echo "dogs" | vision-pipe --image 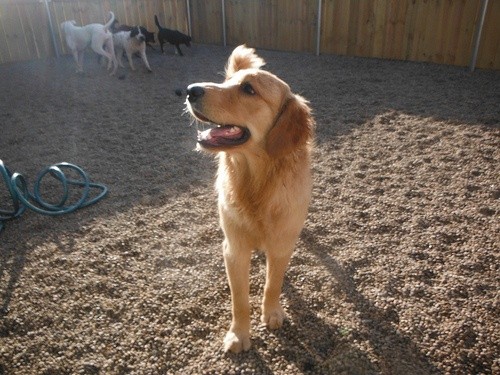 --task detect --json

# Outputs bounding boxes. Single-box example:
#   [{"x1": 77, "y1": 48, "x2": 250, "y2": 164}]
[
  {"x1": 61, "y1": 10, "x2": 193, "y2": 75},
  {"x1": 184, "y1": 44, "x2": 312, "y2": 353}
]
